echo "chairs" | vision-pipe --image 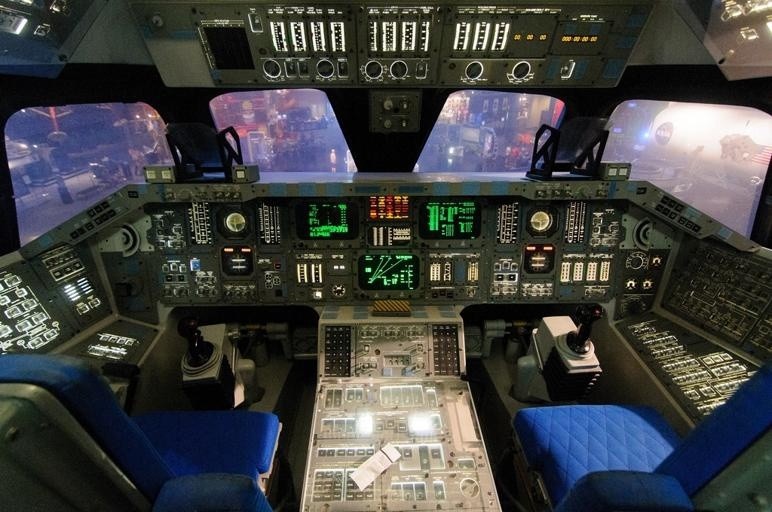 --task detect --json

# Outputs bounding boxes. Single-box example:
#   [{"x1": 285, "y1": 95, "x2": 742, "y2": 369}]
[
  {"x1": 1, "y1": 352, "x2": 299, "y2": 511},
  {"x1": 509, "y1": 362, "x2": 770, "y2": 511}
]
